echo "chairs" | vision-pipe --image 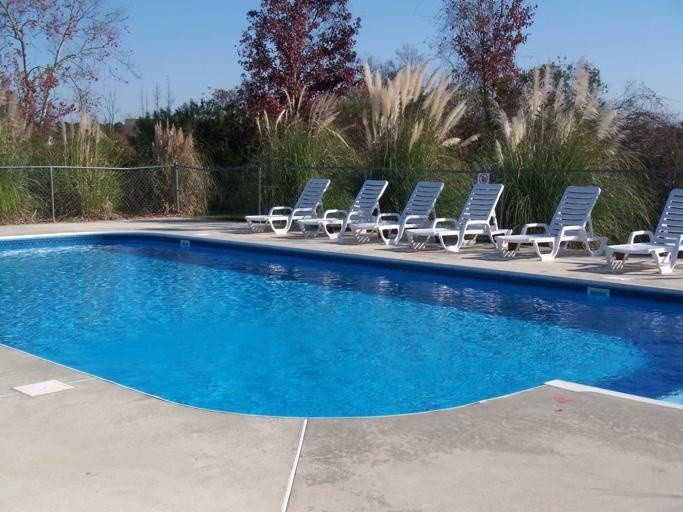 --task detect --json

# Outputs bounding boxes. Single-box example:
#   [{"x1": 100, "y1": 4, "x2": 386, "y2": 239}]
[
  {"x1": 245, "y1": 179, "x2": 331, "y2": 235},
  {"x1": 405, "y1": 184, "x2": 512, "y2": 254},
  {"x1": 603, "y1": 189, "x2": 683, "y2": 275},
  {"x1": 298, "y1": 180, "x2": 388, "y2": 240},
  {"x1": 350, "y1": 181, "x2": 445, "y2": 246},
  {"x1": 494, "y1": 186, "x2": 608, "y2": 262}
]
